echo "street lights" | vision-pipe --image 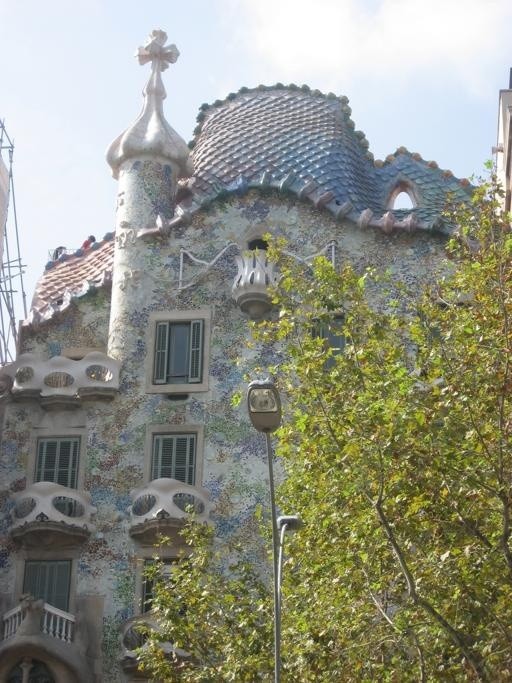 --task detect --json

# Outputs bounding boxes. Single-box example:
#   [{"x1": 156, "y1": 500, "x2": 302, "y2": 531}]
[{"x1": 246, "y1": 379, "x2": 304, "y2": 683}]
